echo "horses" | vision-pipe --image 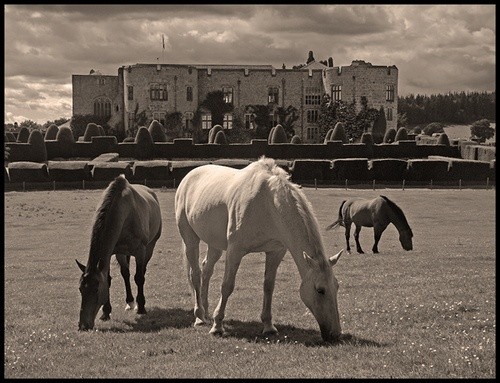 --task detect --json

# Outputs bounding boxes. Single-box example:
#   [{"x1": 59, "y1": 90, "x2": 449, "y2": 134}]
[
  {"x1": 74, "y1": 173, "x2": 162, "y2": 330},
  {"x1": 325, "y1": 194, "x2": 413, "y2": 253},
  {"x1": 174, "y1": 154, "x2": 343, "y2": 343}
]
